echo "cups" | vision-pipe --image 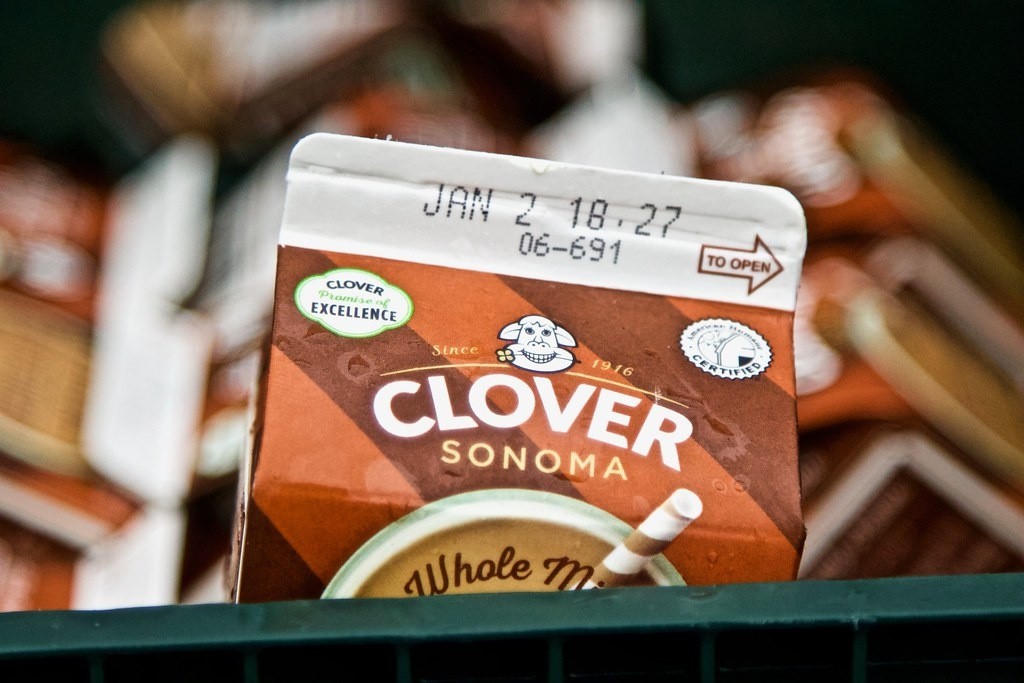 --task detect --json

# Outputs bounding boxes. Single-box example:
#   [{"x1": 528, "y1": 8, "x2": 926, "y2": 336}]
[{"x1": 322, "y1": 492, "x2": 688, "y2": 612}]
